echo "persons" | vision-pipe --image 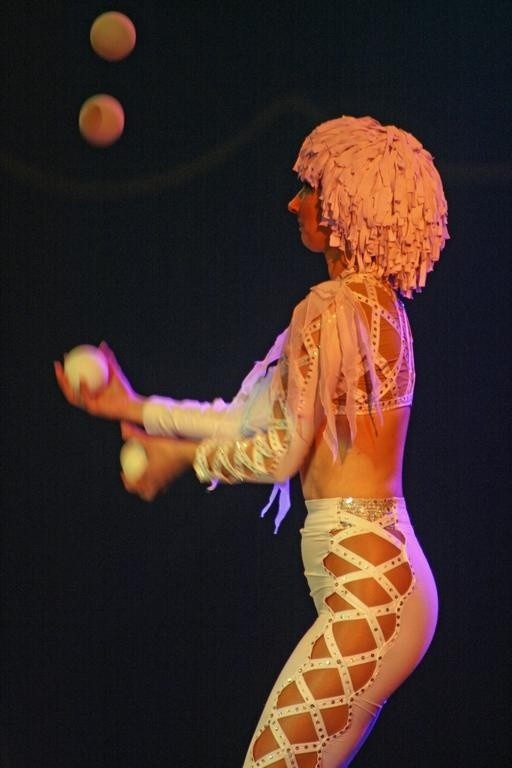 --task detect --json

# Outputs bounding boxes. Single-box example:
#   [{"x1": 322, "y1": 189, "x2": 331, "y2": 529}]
[{"x1": 51, "y1": 114, "x2": 450, "y2": 768}]
